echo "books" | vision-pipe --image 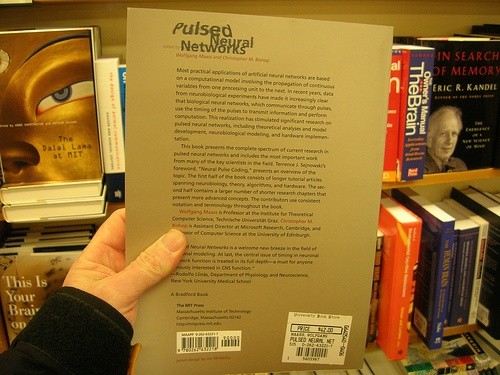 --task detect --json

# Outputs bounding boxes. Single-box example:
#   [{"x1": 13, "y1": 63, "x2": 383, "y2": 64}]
[
  {"x1": 0, "y1": 218, "x2": 97, "y2": 353},
  {"x1": 258, "y1": 22, "x2": 500, "y2": 374},
  {"x1": 125, "y1": 3, "x2": 396, "y2": 375},
  {"x1": 2, "y1": 25, "x2": 128, "y2": 223}
]
